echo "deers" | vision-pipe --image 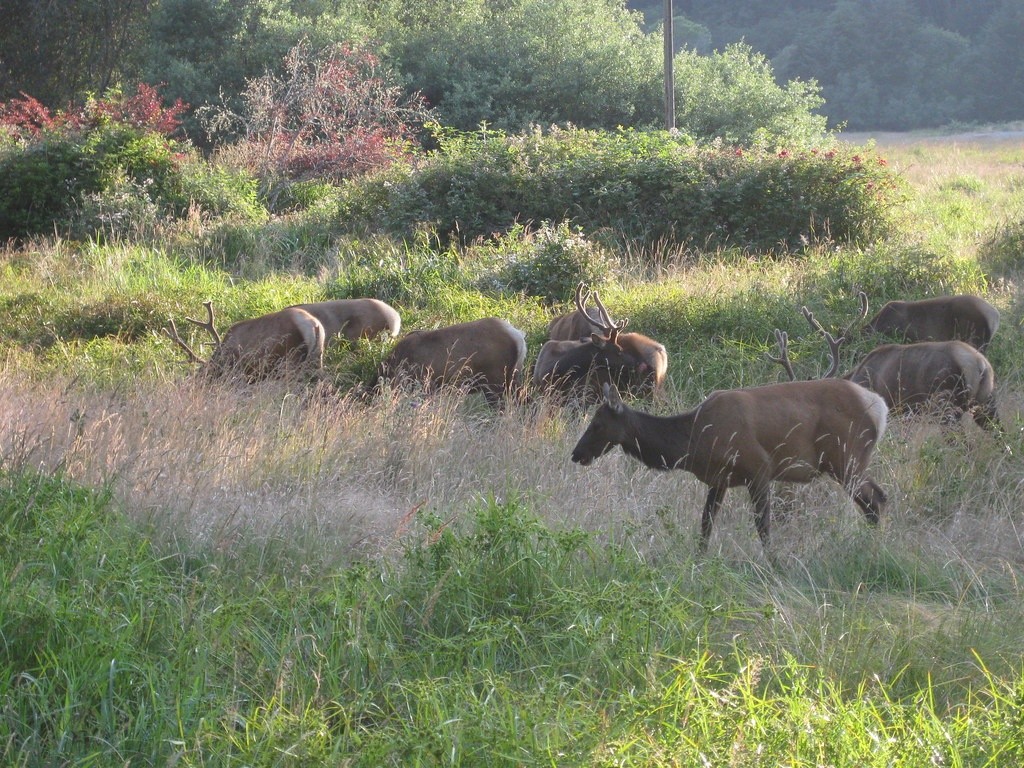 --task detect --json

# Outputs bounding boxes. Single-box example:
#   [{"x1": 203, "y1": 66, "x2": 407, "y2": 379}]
[
  {"x1": 802, "y1": 292, "x2": 1000, "y2": 358},
  {"x1": 361, "y1": 316, "x2": 528, "y2": 415},
  {"x1": 571, "y1": 382, "x2": 888, "y2": 562},
  {"x1": 162, "y1": 298, "x2": 401, "y2": 384},
  {"x1": 762, "y1": 328, "x2": 1005, "y2": 448},
  {"x1": 533, "y1": 281, "x2": 668, "y2": 412}
]
